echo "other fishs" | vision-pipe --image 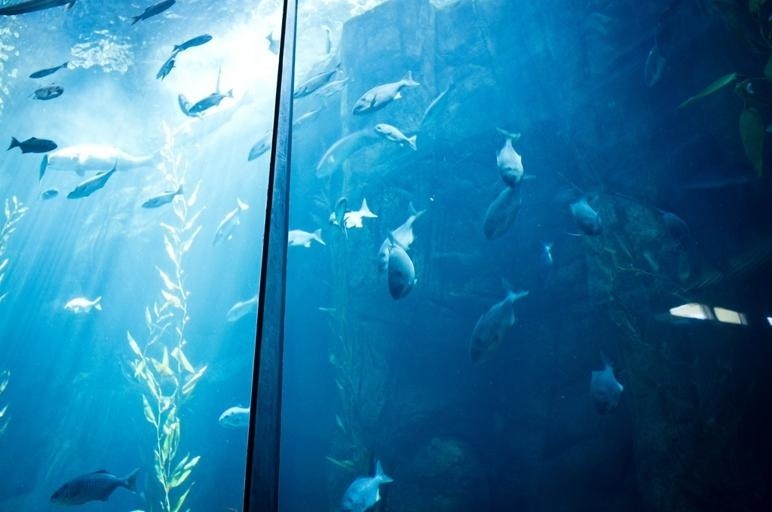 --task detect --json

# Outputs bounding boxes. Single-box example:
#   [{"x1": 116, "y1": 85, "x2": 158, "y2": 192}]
[{"x1": 1, "y1": 0, "x2": 455, "y2": 300}]
